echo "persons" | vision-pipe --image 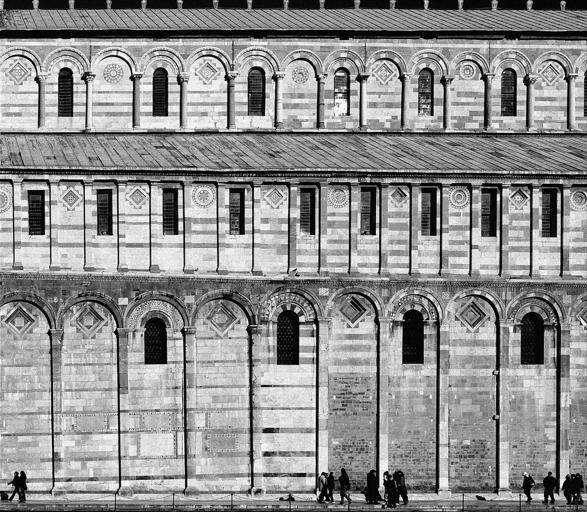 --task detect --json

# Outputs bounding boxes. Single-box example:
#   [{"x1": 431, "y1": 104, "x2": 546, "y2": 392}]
[
  {"x1": 316, "y1": 467, "x2": 410, "y2": 510},
  {"x1": 519, "y1": 470, "x2": 585, "y2": 507},
  {"x1": 5, "y1": 471, "x2": 22, "y2": 503},
  {"x1": 17, "y1": 469, "x2": 28, "y2": 504}
]
